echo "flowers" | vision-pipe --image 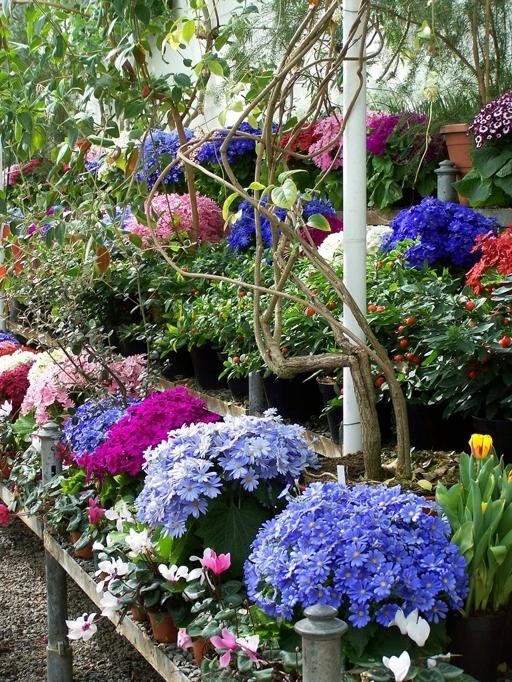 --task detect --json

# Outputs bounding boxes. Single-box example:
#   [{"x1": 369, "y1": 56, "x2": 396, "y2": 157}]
[{"x1": 0, "y1": 90, "x2": 512, "y2": 682}]
